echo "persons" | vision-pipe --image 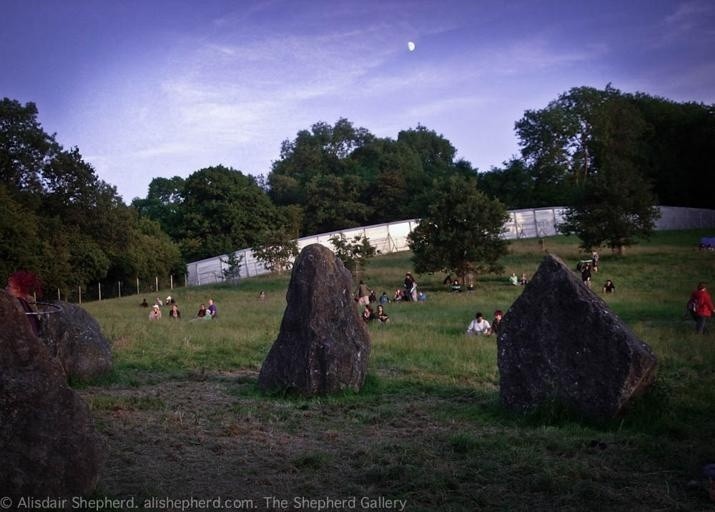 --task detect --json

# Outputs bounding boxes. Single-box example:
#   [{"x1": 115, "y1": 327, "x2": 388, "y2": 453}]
[
  {"x1": 463, "y1": 312, "x2": 491, "y2": 335},
  {"x1": 375, "y1": 305, "x2": 392, "y2": 324},
  {"x1": 361, "y1": 305, "x2": 375, "y2": 323},
  {"x1": 347, "y1": 267, "x2": 473, "y2": 305},
  {"x1": 686, "y1": 281, "x2": 715, "y2": 335},
  {"x1": 575, "y1": 249, "x2": 599, "y2": 292},
  {"x1": 507, "y1": 272, "x2": 522, "y2": 285},
  {"x1": 603, "y1": 279, "x2": 616, "y2": 294},
  {"x1": 520, "y1": 273, "x2": 529, "y2": 285},
  {"x1": 491, "y1": 310, "x2": 503, "y2": 337},
  {"x1": 260, "y1": 290, "x2": 265, "y2": 298},
  {"x1": 699, "y1": 242, "x2": 705, "y2": 251},
  {"x1": 139, "y1": 294, "x2": 217, "y2": 321},
  {"x1": 708, "y1": 243, "x2": 714, "y2": 251}
]
